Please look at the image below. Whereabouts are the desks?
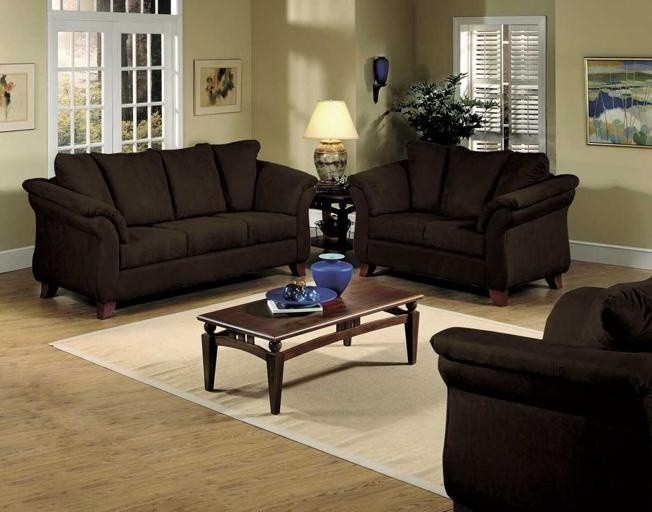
[310,195,356,260]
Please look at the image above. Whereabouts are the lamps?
[371,57,390,103]
[302,100,361,186]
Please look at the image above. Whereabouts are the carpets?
[49,280,543,502]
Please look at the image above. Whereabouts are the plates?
[266,286,338,304]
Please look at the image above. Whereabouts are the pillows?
[54,139,260,227]
[405,140,550,219]
[582,277,651,353]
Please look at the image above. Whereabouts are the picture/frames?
[0,62,38,132]
[582,55,652,150]
[191,59,243,116]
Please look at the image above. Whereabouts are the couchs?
[347,145,578,305]
[22,139,318,319]
[429,277,651,512]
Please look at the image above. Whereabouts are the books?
[267,300,324,315]
